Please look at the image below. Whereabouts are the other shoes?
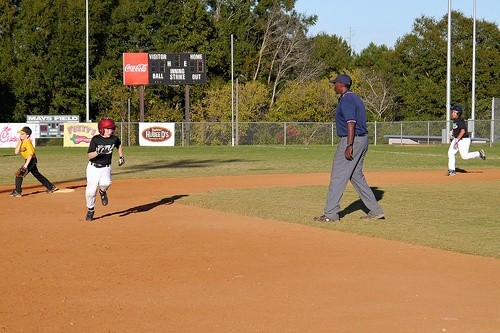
[479,149,486,160]
[313,215,335,222]
[445,169,456,176]
[9,190,22,197]
[47,186,58,194]
[359,211,385,221]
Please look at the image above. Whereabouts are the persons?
[446,106,486,176]
[85,117,125,221]
[313,75,385,222]
[8,127,58,197]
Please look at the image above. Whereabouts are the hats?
[329,74,353,85]
[450,106,462,111]
[17,126,32,135]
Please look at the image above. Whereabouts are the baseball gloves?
[96,146,110,155]
[16,168,28,178]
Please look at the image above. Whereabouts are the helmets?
[98,118,118,136]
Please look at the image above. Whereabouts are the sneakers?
[86,207,95,222]
[99,189,108,206]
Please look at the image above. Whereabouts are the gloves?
[95,147,110,154]
[118,156,125,167]
[452,138,459,149]
[449,130,454,139]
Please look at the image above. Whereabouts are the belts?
[338,133,367,138]
[91,162,109,168]
